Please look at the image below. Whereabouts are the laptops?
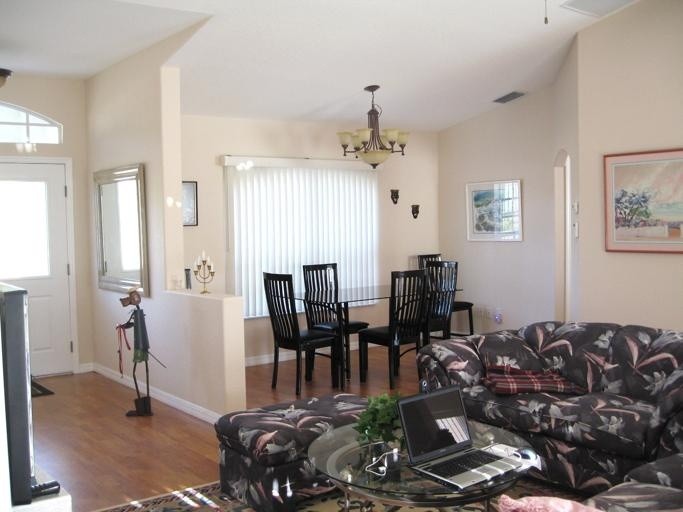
[395,383,522,492]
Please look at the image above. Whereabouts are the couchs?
[414,319,682,510]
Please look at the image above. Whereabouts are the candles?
[192,251,216,275]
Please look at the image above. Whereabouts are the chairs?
[260,251,473,397]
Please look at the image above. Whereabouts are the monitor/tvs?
[0,281,37,505]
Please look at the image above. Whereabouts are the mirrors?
[94,164,148,297]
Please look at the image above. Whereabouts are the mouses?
[512,447,538,462]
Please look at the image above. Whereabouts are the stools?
[214,391,400,512]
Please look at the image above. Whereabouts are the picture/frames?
[180,180,199,227]
[466,179,523,243]
[603,151,682,256]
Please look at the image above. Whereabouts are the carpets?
[31,381,54,399]
[99,450,594,512]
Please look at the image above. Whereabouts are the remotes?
[32,481,62,498]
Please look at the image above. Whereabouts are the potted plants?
[356,393,401,444]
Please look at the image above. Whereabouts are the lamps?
[338,85,411,168]
